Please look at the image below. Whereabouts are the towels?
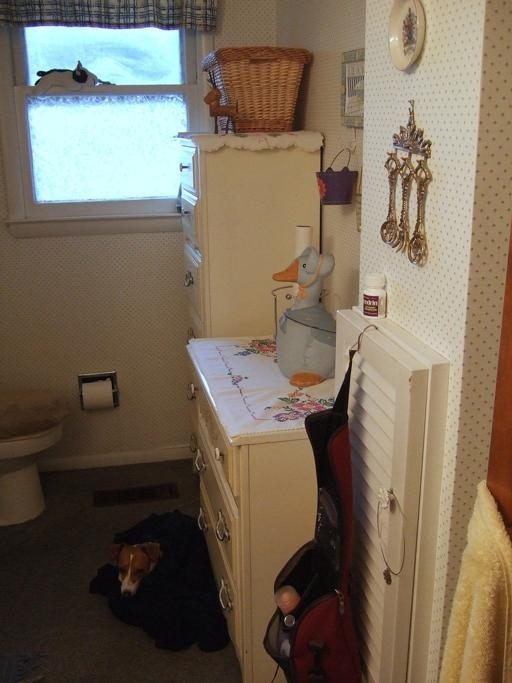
[438,479,511,682]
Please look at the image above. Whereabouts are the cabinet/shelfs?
[185,338,334,682]
[175,132,325,346]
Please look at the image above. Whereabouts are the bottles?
[276,587,300,615]
[362,273,387,317]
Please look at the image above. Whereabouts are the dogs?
[110,541,162,597]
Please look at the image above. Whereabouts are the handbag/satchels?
[263,410,363,681]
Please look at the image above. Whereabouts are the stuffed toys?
[270,245,335,389]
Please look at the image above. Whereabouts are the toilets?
[0,392,62,528]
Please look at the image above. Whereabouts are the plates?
[387,0,425,71]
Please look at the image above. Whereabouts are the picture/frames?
[341,48,364,128]
